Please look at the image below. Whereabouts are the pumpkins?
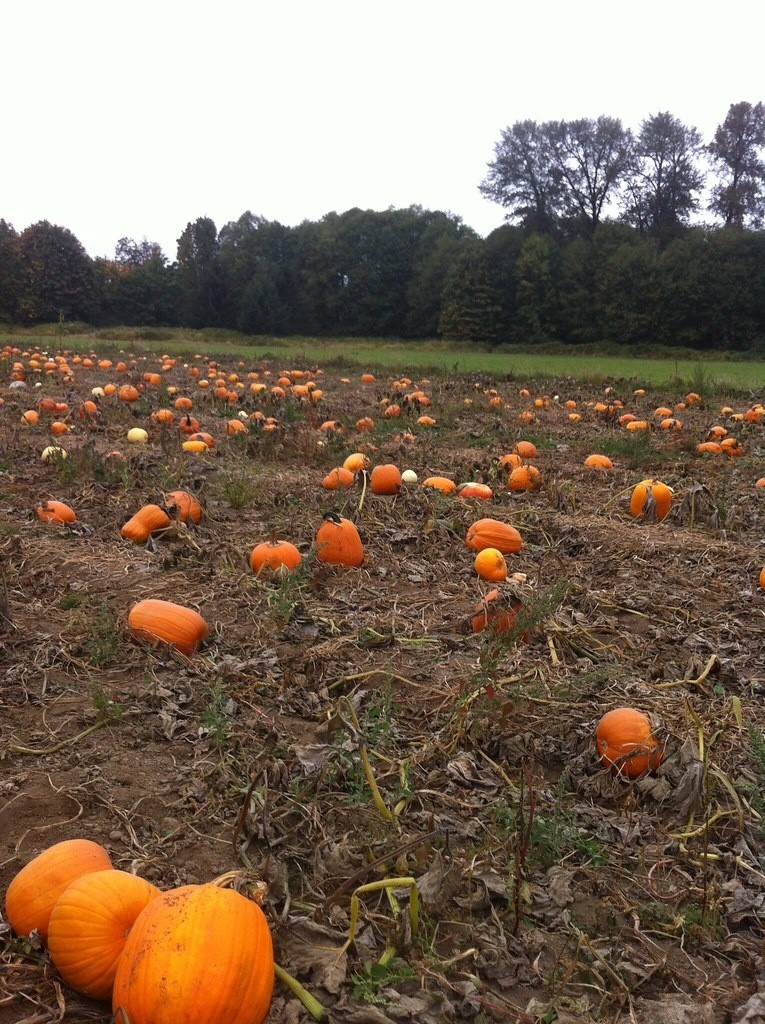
[597,708,662,779]
[472,589,533,644]
[5,838,115,946]
[129,598,208,655]
[0,346,765,589]
[48,870,162,998]
[112,870,274,1023]
